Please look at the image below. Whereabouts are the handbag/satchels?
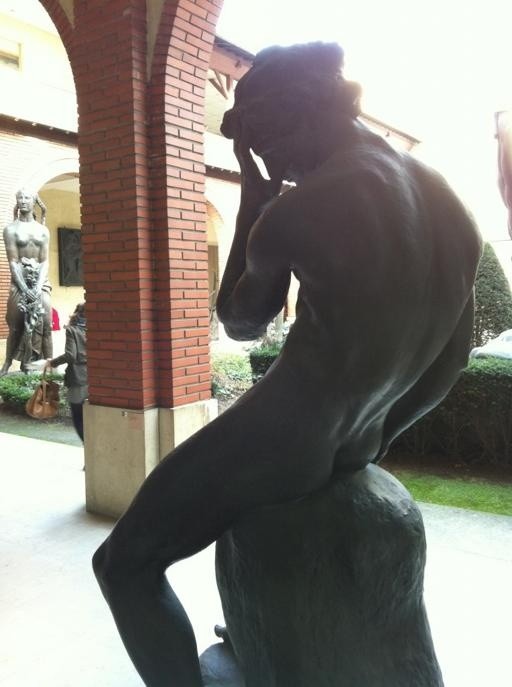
[26,381,61,419]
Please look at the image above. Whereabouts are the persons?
[47,304,87,442]
[50,307,60,331]
[1,186,54,377]
[89,39,487,686]
